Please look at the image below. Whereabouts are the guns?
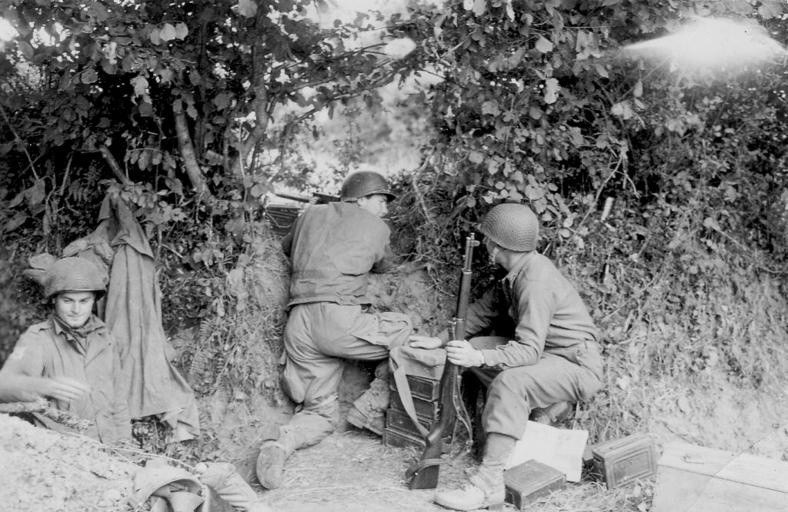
[276,192,340,204]
[409,232,481,489]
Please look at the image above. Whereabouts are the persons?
[254,170,413,490]
[0,257,132,451]
[406,202,603,512]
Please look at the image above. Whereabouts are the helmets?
[476,203,539,253]
[43,257,107,302]
[341,172,399,202]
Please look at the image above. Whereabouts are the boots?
[534,402,572,427]
[346,378,392,437]
[256,433,303,492]
[434,433,516,512]
